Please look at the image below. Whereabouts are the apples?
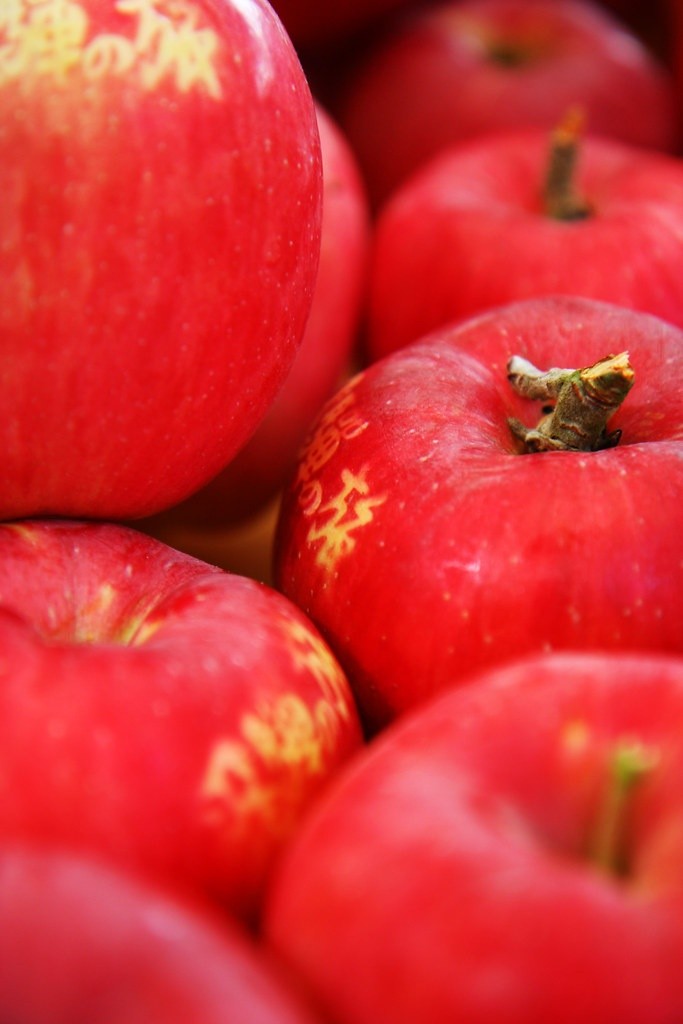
[0,0,683,1024]
